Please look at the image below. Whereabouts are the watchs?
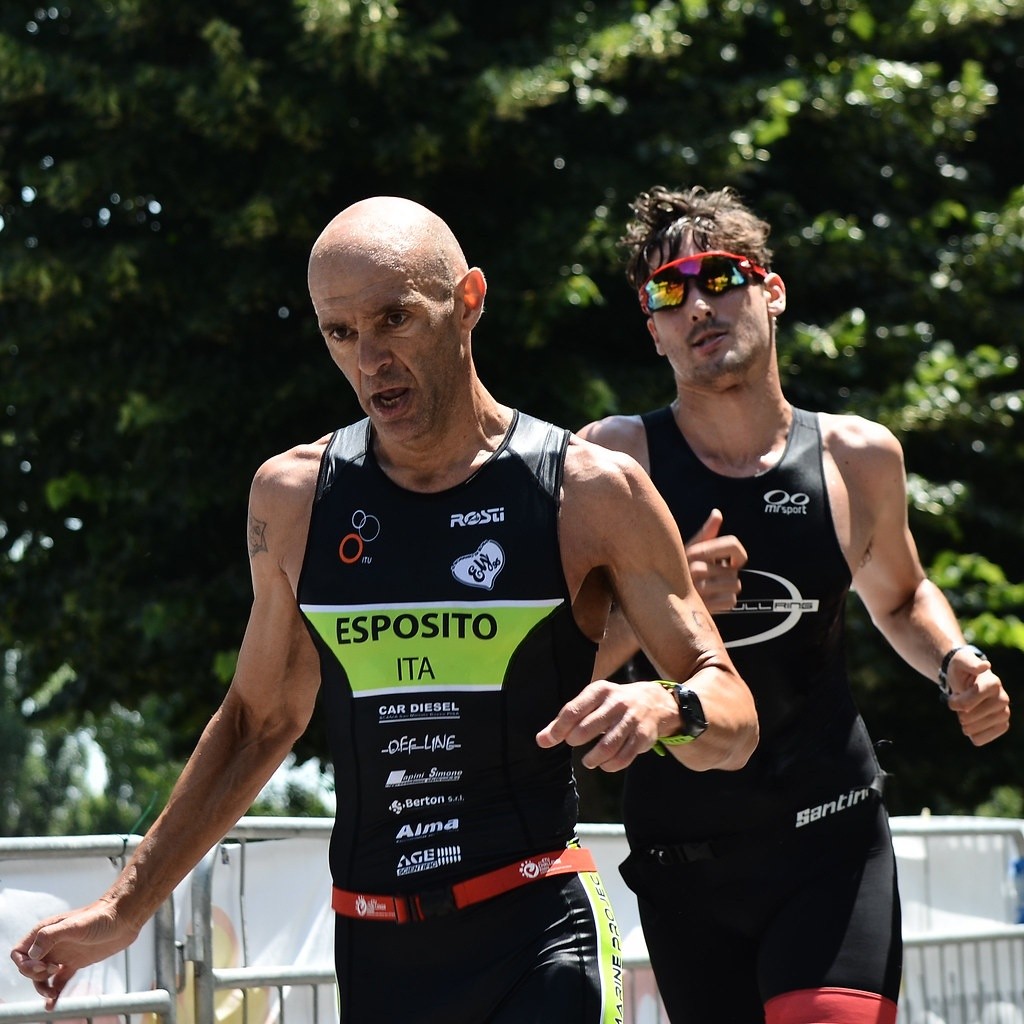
[652,680,708,756]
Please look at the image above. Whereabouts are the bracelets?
[938,646,987,697]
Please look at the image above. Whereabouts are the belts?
[333,849,598,925]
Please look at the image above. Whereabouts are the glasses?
[638,251,767,314]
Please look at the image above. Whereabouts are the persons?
[10,197,758,1024]
[568,189,1011,1024]
